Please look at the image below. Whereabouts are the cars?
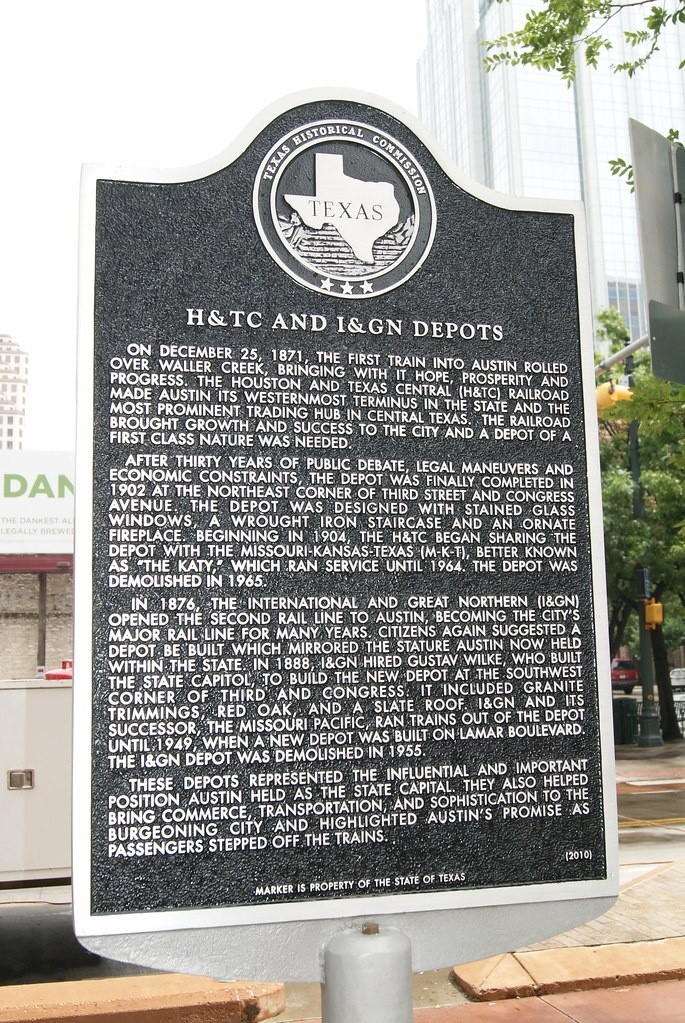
[669,667,685,692]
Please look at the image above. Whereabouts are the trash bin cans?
[613,696,638,743]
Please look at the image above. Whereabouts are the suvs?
[610,658,639,695]
